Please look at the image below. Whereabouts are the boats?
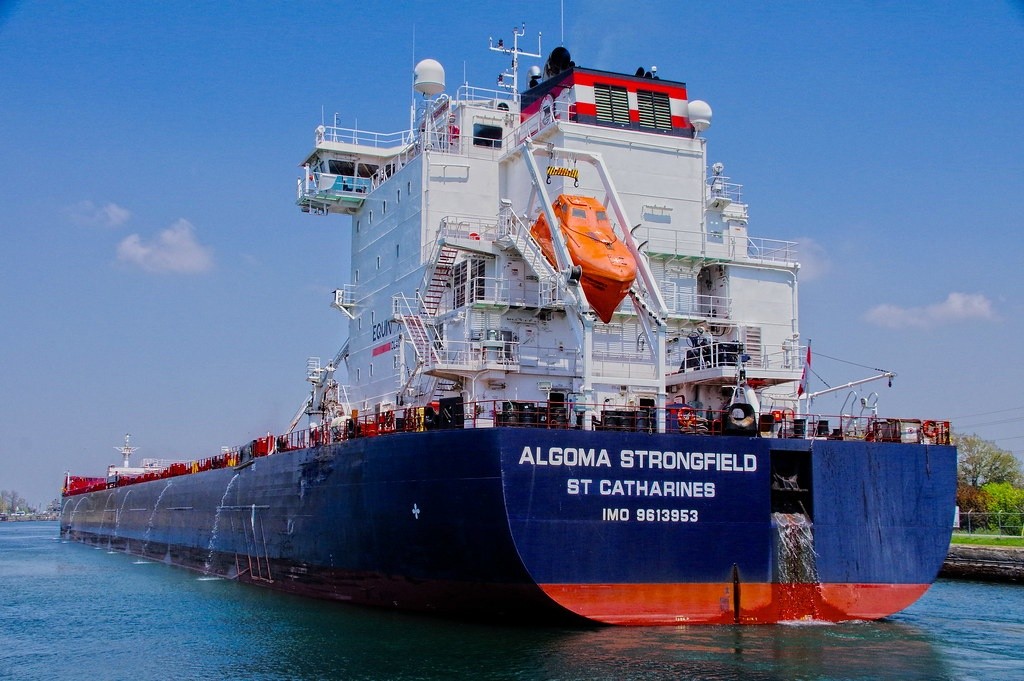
[59,0,960,628]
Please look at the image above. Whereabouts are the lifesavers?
[923,420,939,437]
[677,407,695,426]
[386,410,394,427]
